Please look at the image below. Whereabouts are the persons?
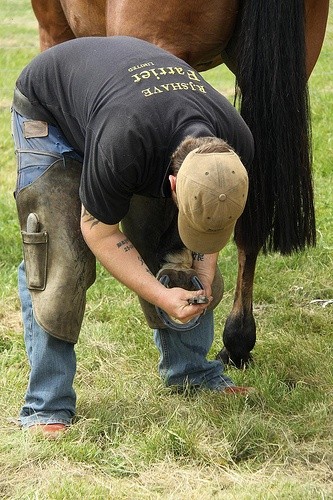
[7,34,263,445]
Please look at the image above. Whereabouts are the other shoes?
[184,383,258,408]
[21,418,74,442]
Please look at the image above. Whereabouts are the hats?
[177,145,250,254]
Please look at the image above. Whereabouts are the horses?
[30,0,328,370]
